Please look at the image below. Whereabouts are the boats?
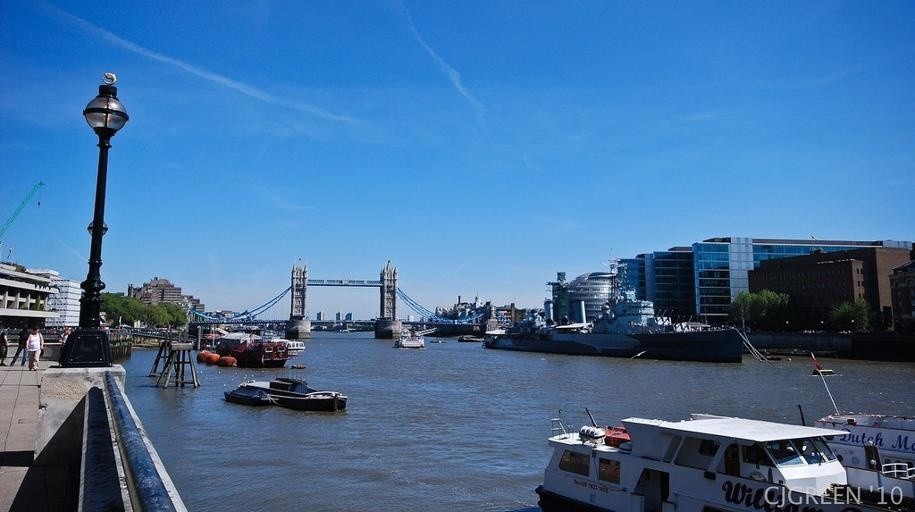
[224,377,346,412]
[392,330,424,348]
[187,319,306,368]
[813,368,834,375]
[744,348,838,361]
[458,336,485,341]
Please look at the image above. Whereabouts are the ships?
[481,248,743,362]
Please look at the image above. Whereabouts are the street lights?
[57,71,130,331]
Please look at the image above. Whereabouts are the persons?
[10,325,30,366]
[26,327,44,370]
[0,325,11,366]
[5,327,76,343]
[99,323,123,342]
